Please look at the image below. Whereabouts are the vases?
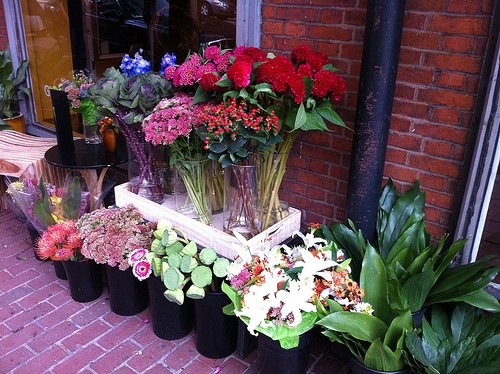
[27,228,51,261]
[257,332,312,374]
[62,260,104,303]
[151,274,195,341]
[175,158,213,227]
[256,151,289,229]
[128,141,165,204]
[196,292,238,358]
[349,355,411,374]
[106,265,151,317]
[55,262,68,280]
[83,116,103,144]
[223,163,260,239]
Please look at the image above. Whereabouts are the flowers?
[6,40,500,374]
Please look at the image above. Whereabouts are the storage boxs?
[113,183,302,261]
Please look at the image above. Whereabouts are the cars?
[90,0,236,60]
[199,0,237,20]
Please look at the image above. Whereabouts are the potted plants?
[0,50,34,136]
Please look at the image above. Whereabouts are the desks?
[45,139,135,209]
